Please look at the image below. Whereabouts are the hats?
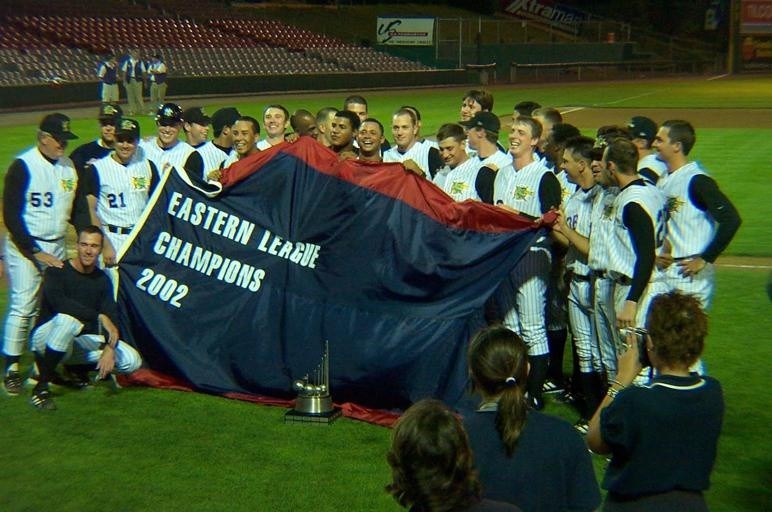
[97,101,123,120]
[105,53,115,59]
[212,107,240,125]
[624,116,658,141]
[180,106,212,124]
[458,111,500,132]
[152,54,160,59]
[113,119,139,138]
[40,114,78,140]
[154,102,183,126]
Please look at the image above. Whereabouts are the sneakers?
[149,110,156,116]
[542,380,566,394]
[31,389,51,408]
[1,371,22,396]
[128,109,135,117]
[66,371,95,391]
[552,391,579,403]
[137,109,146,115]
[28,362,66,386]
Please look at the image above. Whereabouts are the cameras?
[618,327,648,355]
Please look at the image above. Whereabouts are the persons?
[121,49,147,116]
[453,326,603,512]
[98,53,121,106]
[652,119,742,376]
[31,225,143,410]
[587,288,724,511]
[2,112,90,397]
[68,89,670,434]
[147,55,167,115]
[385,399,520,510]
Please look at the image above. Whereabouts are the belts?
[573,273,589,282]
[104,82,116,85]
[616,278,633,286]
[673,258,686,262]
[107,225,132,234]
[150,80,157,83]
[129,77,135,79]
[597,272,606,278]
[29,235,66,244]
